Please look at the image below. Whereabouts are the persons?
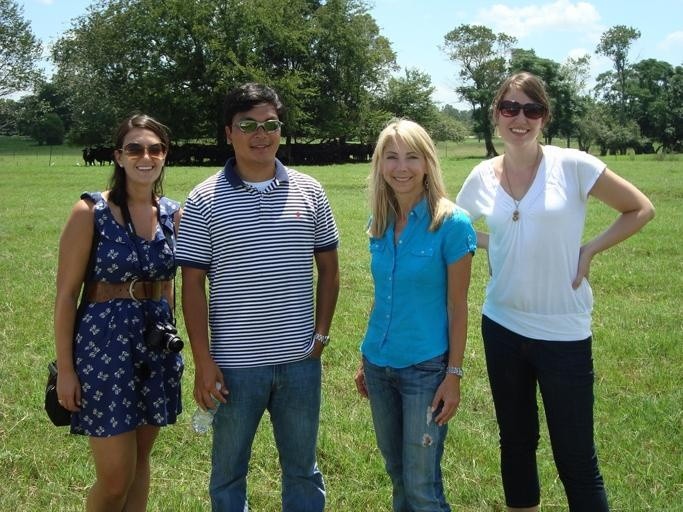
[454,73,654,511]
[175,85,338,512]
[54,112,182,512]
[351,119,478,511]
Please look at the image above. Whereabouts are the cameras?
[145,320,184,353]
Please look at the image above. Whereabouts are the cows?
[82,142,376,166]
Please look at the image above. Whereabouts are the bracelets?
[444,366,464,377]
[314,332,332,346]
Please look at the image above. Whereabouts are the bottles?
[191,381,220,434]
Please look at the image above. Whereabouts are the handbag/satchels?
[44,360,72,426]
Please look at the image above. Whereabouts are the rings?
[58,398,65,404]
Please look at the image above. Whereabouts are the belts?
[84,279,172,304]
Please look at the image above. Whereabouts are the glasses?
[234,119,284,133]
[498,99,548,119]
[119,142,168,160]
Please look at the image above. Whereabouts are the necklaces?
[502,146,541,221]
[237,166,276,180]
